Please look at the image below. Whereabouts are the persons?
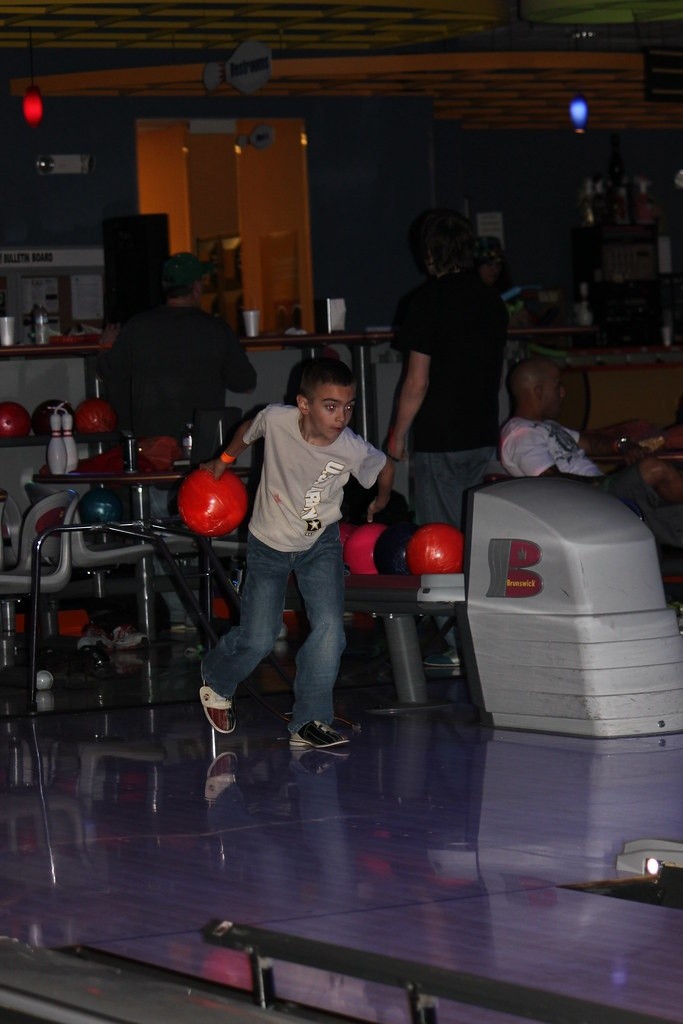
[94,252,257,634]
[498,356,683,552]
[472,237,523,307]
[386,209,510,669]
[203,747,352,918]
[197,354,393,748]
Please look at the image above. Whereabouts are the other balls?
[0,402,32,439]
[80,488,125,526]
[32,399,75,435]
[74,398,117,432]
[405,523,465,577]
[178,464,250,538]
[374,521,418,576]
[343,524,386,575]
[337,523,355,546]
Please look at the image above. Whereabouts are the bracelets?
[219,451,236,465]
[622,441,635,453]
[617,437,629,449]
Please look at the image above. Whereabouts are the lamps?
[567,88,590,135]
[22,28,45,130]
[35,152,95,177]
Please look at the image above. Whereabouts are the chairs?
[25,480,158,602]
[0,488,22,633]
[0,488,79,640]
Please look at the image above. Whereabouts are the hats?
[469,237,504,261]
[161,252,217,286]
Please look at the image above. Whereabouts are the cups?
[244,311,260,337]
[0,316,15,346]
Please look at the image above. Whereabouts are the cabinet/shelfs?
[572,223,664,346]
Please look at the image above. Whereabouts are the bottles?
[36,303,49,345]
[183,423,195,459]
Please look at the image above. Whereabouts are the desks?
[0,324,593,445]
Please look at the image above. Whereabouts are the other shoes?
[424,648,461,667]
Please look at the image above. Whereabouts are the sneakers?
[112,624,149,648]
[200,661,236,734]
[76,624,113,651]
[289,722,350,749]
